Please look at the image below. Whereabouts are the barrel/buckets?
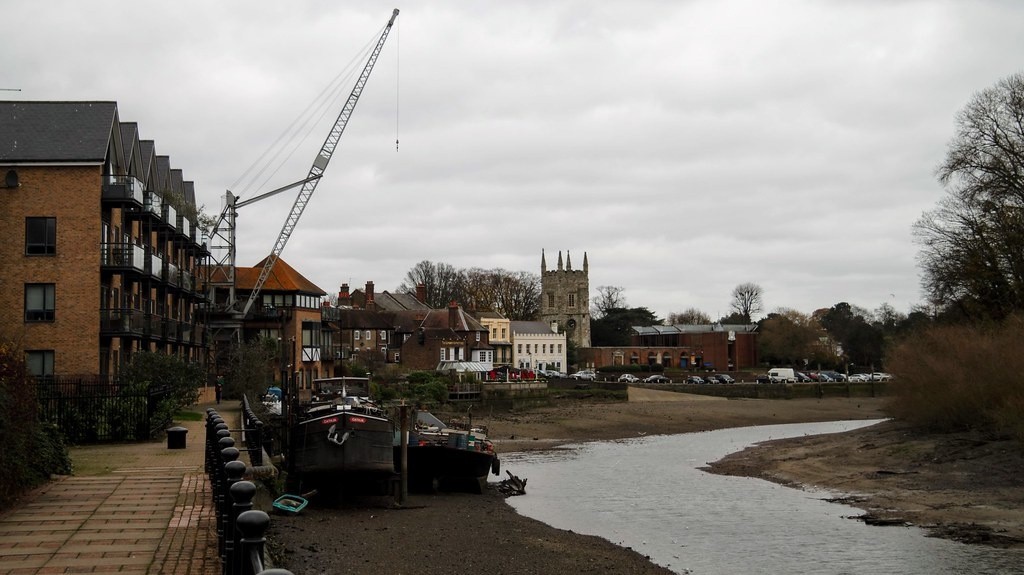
[408,433,418,447]
[447,432,475,450]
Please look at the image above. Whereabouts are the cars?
[533,370,569,379]
[755,375,778,384]
[685,376,704,384]
[820,374,833,382]
[795,372,811,382]
[842,373,877,383]
[619,375,640,383]
[643,375,670,384]
[703,376,720,384]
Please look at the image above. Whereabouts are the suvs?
[571,371,597,381]
[715,374,735,384]
[870,373,889,381]
[801,371,819,382]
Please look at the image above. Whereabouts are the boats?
[393,404,495,490]
[263,388,282,417]
[282,301,394,499]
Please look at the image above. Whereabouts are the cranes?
[205,7,398,381]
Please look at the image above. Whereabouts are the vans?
[820,371,846,382]
[768,368,795,383]
